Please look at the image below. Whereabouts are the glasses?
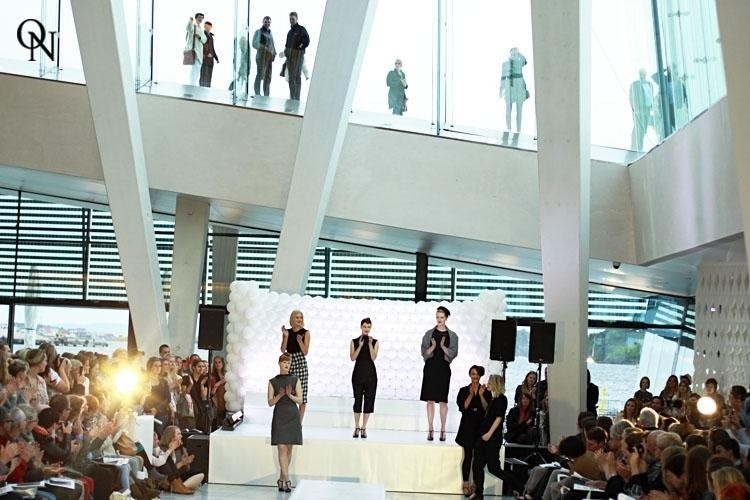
[7,419,15,423]
[395,62,401,64]
[67,408,73,412]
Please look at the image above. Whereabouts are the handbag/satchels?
[183,24,195,65]
[525,84,529,99]
[129,471,160,500]
[115,432,137,456]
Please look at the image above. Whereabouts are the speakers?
[529,322,556,364]
[490,319,517,362]
[198,307,225,351]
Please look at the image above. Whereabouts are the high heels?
[353,427,360,437]
[467,491,484,500]
[285,480,292,493]
[439,431,446,441]
[427,430,435,441]
[277,479,284,492]
[361,429,367,438]
[463,486,472,498]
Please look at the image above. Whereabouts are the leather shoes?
[178,478,195,491]
[158,480,171,491]
[171,479,195,495]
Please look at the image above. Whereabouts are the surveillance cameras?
[613,262,622,269]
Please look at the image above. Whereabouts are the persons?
[613,373,750,421]
[464,374,524,500]
[127,343,227,432]
[629,69,653,152]
[0,340,127,410]
[350,318,378,437]
[499,48,530,135]
[652,63,689,140]
[536,367,548,400]
[455,366,490,496]
[252,16,277,95]
[0,407,162,499]
[586,370,599,414]
[507,393,536,443]
[200,22,219,87]
[183,13,207,88]
[268,353,303,492]
[279,12,309,98]
[514,372,537,405]
[281,311,311,422]
[386,60,409,116]
[153,434,195,495]
[159,425,205,489]
[419,306,458,441]
[512,412,750,500]
